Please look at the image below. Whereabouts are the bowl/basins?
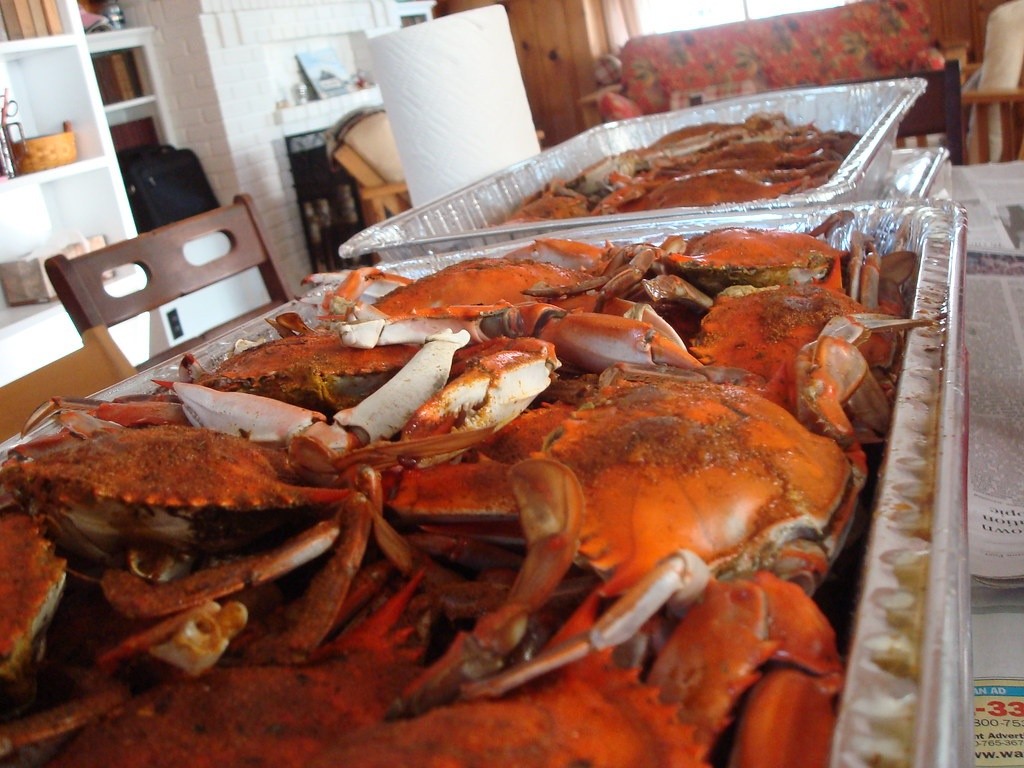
[13,120,76,173]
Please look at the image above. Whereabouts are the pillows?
[601,91,641,119]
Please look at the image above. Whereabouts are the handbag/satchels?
[125,144,222,236]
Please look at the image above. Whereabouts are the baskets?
[5,120,78,174]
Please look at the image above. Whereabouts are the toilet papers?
[359,4,543,211]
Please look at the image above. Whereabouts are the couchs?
[597,0,972,123]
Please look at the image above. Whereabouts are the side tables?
[579,84,622,128]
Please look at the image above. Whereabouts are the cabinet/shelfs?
[0,0,150,386]
[284,126,374,273]
[85,26,180,150]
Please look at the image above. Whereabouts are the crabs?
[0,209,943,768]
[486,110,865,227]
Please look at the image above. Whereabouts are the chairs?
[824,58,964,165]
[0,325,137,444]
[44,193,291,372]
[325,105,546,263]
